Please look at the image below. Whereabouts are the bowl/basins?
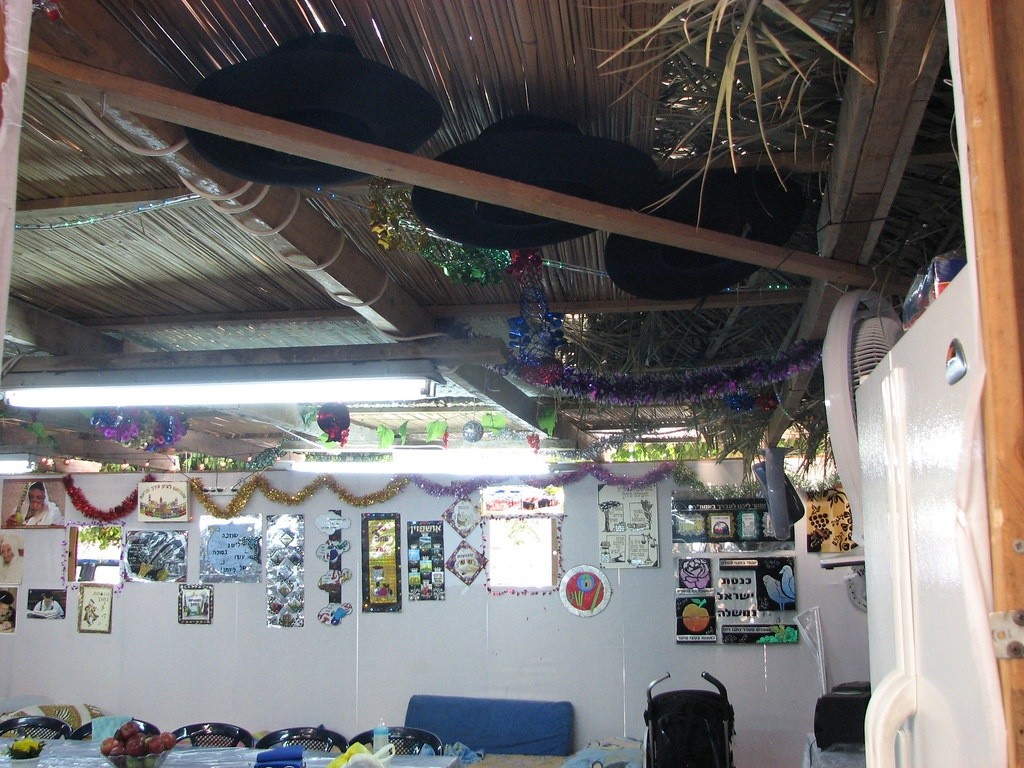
[98,749,173,768]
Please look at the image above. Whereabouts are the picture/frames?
[361,512,402,613]
[178,583,214,624]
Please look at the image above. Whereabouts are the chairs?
[404,694,573,754]
[0,714,445,754]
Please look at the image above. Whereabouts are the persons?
[0,590,16,633]
[6,481,65,526]
[0,534,22,580]
[34,591,64,615]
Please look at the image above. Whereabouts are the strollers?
[642,673,736,768]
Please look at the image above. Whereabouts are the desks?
[0,738,459,768]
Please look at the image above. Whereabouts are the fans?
[823,289,905,564]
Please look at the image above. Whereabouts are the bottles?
[373,718,389,768]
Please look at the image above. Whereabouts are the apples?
[101,722,176,768]
